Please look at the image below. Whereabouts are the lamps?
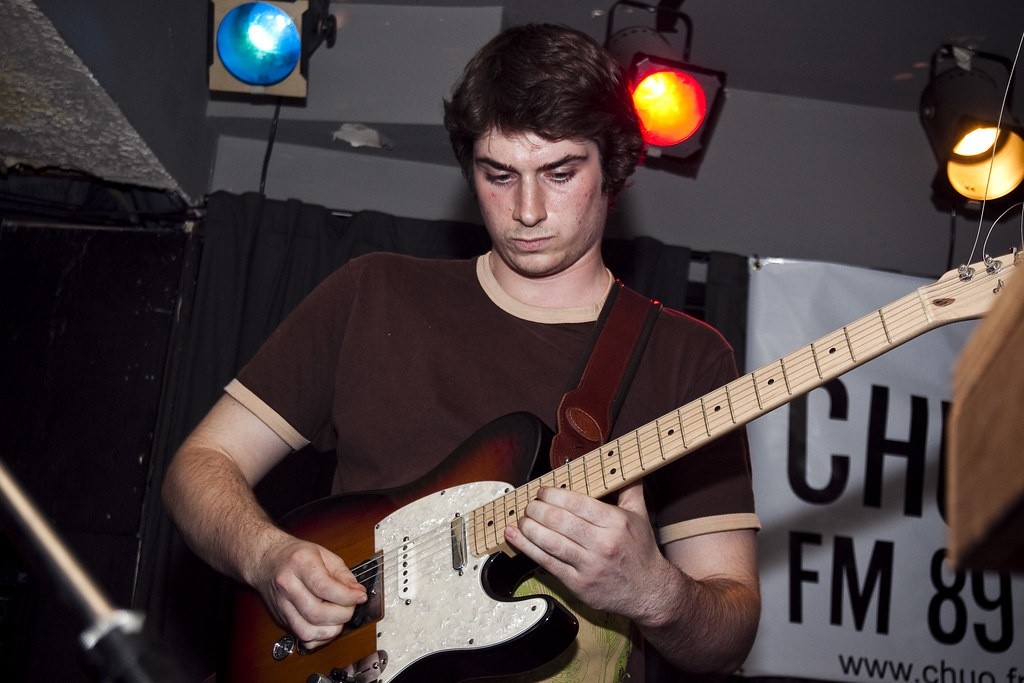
[918,43,1024,221]
[603,0,728,180]
[208,0,336,98]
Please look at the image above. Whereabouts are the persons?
[164,24,763,683]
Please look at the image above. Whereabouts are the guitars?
[235,245,1018,683]
